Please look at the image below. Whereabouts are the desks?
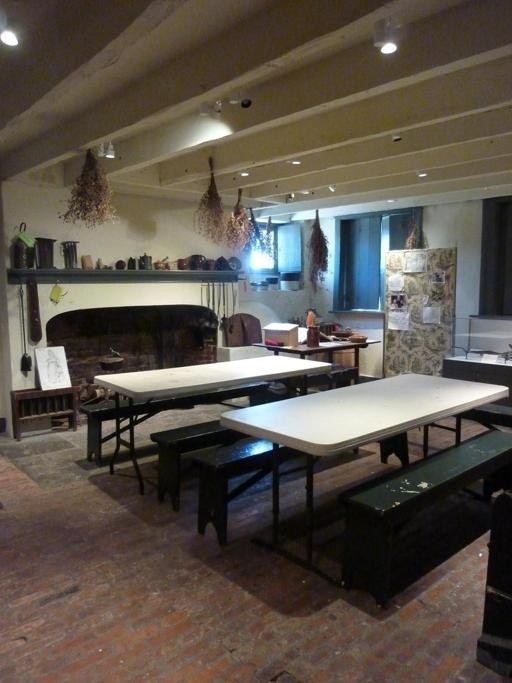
[251,337,380,395]
[218,371,511,583]
[92,354,334,500]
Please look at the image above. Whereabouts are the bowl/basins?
[154,261,168,270]
[348,335,368,343]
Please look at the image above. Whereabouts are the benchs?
[76,384,512,595]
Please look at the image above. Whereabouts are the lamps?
[372,15,399,57]
[96,141,116,158]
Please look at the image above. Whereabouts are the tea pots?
[137,252,153,270]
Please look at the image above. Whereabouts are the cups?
[190,254,207,270]
[207,259,216,271]
[176,258,189,269]
